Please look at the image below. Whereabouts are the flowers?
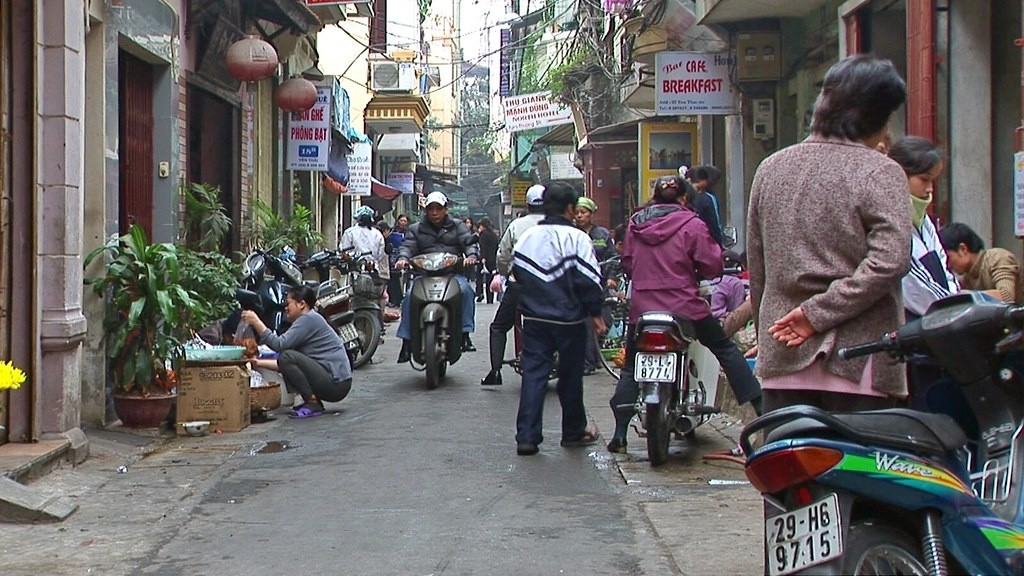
[0,361,27,430]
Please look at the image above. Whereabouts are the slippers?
[561,425,598,448]
[516,442,539,456]
[287,408,322,418]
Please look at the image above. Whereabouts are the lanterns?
[225,35,278,84]
[273,75,318,115]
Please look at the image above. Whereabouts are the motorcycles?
[223,239,386,372]
[498,264,563,381]
[737,289,1023,575]
[384,234,480,389]
[603,226,743,466]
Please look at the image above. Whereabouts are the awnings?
[427,170,458,181]
[427,177,463,195]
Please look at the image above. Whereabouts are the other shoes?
[397,348,410,363]
[607,435,628,453]
[480,370,503,385]
[463,339,476,351]
[477,294,484,302]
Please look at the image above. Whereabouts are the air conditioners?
[370,62,418,90]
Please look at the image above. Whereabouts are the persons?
[198,308,242,345]
[338,54,1019,457]
[241,285,352,418]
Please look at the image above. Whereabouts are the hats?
[425,191,448,206]
[525,184,546,205]
[377,220,388,227]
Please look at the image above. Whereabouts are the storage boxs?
[175,364,252,435]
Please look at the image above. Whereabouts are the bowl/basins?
[181,421,211,437]
[184,345,246,360]
[602,348,620,361]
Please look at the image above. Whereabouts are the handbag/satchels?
[490,274,503,293]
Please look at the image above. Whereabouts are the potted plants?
[83,223,220,428]
